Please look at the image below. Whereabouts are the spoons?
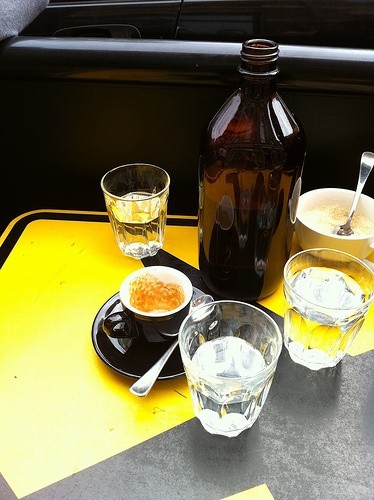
[129,294,215,397]
[334,152,374,236]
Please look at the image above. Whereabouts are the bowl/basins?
[103,265,194,353]
[295,188,374,262]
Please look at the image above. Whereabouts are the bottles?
[197,39,305,302]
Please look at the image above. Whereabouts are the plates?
[91,286,222,382]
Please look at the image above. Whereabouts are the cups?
[178,300,282,438]
[283,248,374,372]
[100,163,170,260]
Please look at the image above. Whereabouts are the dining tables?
[0,209,374,500]
